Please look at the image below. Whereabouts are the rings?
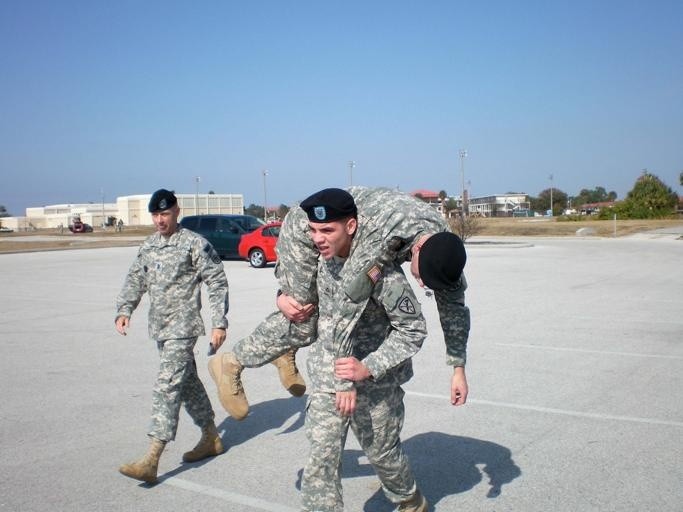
[345,399,350,402]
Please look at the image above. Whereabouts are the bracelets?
[453,365,466,369]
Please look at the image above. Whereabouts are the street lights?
[262,169,268,223]
[194,176,199,215]
[347,160,355,186]
[457,149,467,219]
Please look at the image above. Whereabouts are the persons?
[206,185,469,421]
[276,188,431,510]
[117,218,123,232]
[112,187,229,482]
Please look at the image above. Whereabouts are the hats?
[149,189,177,213]
[300,189,358,223]
[417,232,467,290]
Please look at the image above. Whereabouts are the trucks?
[101,216,116,226]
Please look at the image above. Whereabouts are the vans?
[179,214,267,262]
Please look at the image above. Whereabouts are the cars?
[238,222,282,268]
[0,227,14,233]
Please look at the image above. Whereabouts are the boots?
[118,439,167,484]
[183,424,224,461]
[273,349,307,398]
[208,349,251,420]
